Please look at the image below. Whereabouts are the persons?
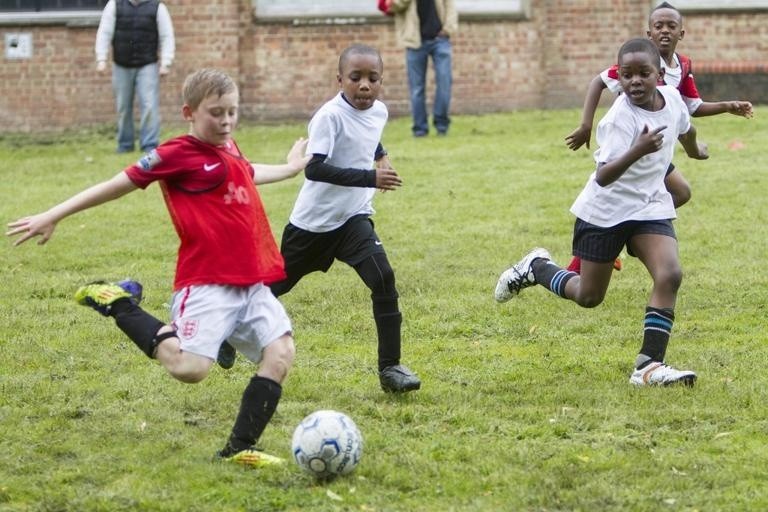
[5,69,314,468]
[564,2,754,273]
[218,45,421,394]
[493,37,710,389]
[95,1,176,152]
[378,1,459,135]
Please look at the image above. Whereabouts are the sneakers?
[567,255,582,272]
[629,361,698,387]
[216,341,236,369]
[212,445,289,469]
[494,247,551,302]
[379,364,421,395]
[73,277,143,317]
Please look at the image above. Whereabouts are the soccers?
[291,410,363,479]
